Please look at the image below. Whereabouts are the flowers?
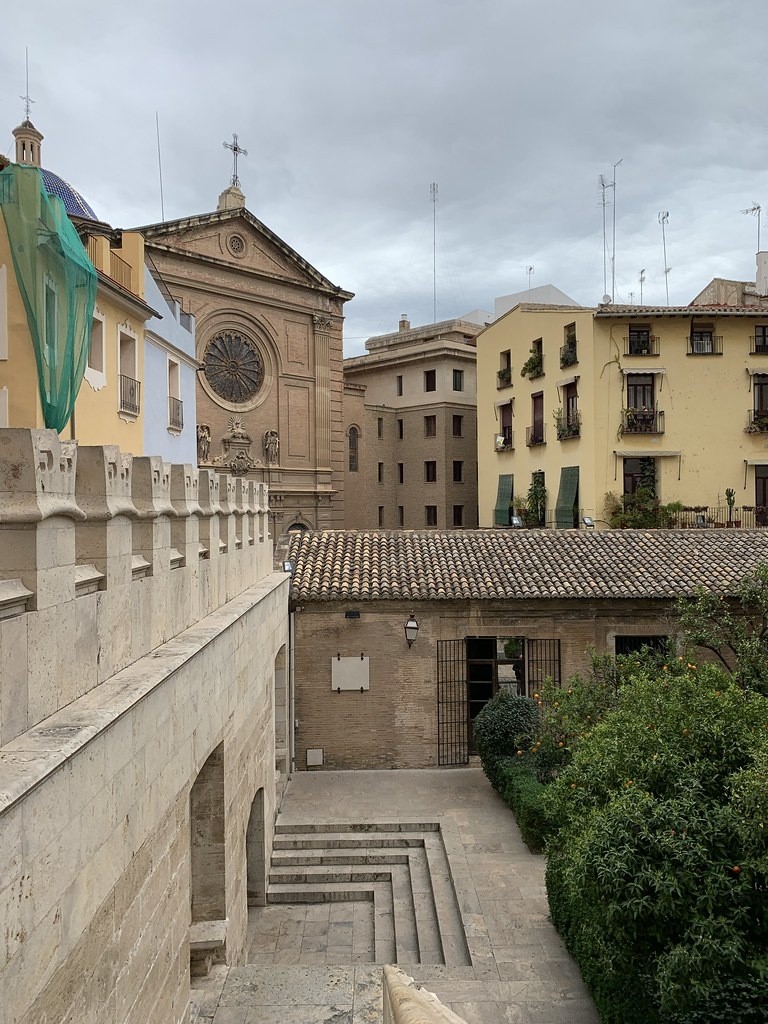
[618,406,654,427]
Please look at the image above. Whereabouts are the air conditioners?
[694,341,712,353]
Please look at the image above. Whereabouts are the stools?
[188,919,227,976]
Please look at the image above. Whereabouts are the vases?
[641,427,646,432]
[651,425,657,432]
[627,428,630,431]
[637,429,640,431]
[632,429,635,432]
[650,335,655,341]
[646,428,650,432]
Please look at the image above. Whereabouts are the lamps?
[282,559,295,573]
[511,516,524,530]
[583,517,595,529]
[344,611,362,620]
[403,610,419,649]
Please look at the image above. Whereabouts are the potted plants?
[751,416,768,431]
[552,407,582,439]
[733,508,742,528]
[724,487,736,528]
[714,491,726,528]
[642,345,648,354]
[497,369,511,386]
[507,495,528,516]
